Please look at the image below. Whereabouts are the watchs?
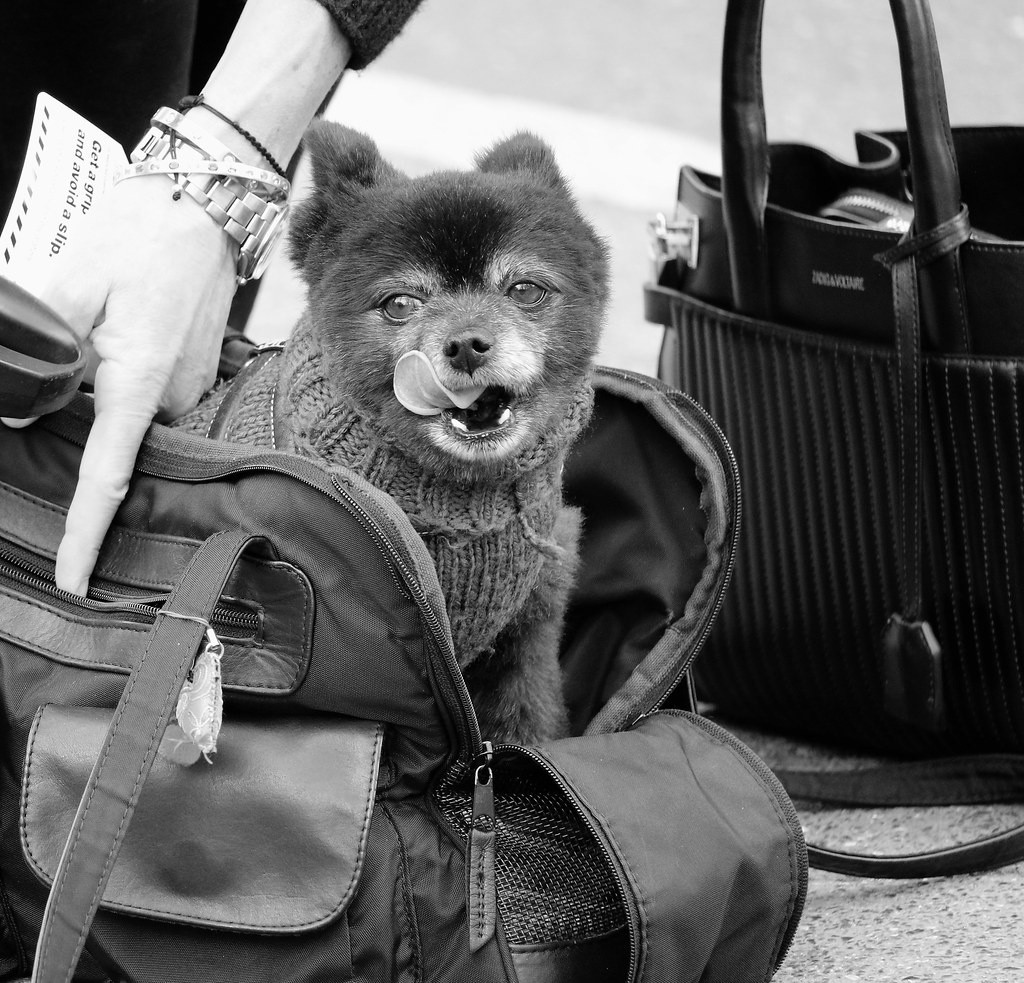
[130,106,289,287]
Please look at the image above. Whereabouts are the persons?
[0,0,426,600]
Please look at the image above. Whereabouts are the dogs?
[153,113,614,756]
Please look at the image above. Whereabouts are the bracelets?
[111,96,291,198]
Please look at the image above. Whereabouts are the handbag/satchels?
[0,329,808,983]
[645,1,1023,763]
